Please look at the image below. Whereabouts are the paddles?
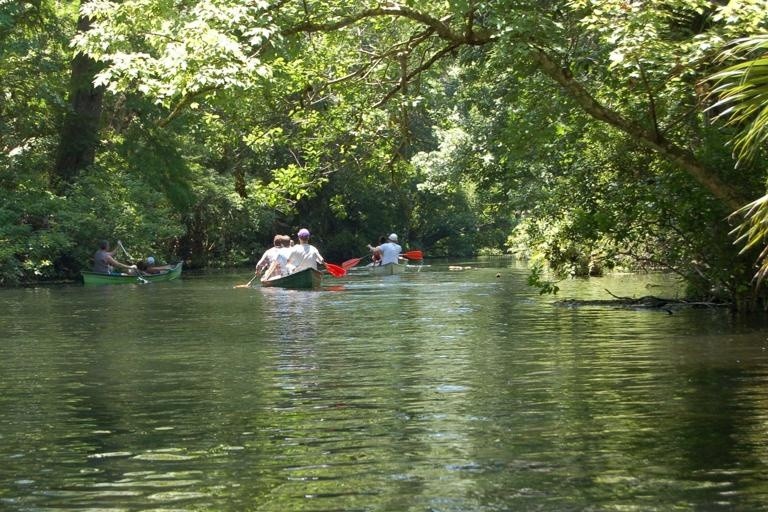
[342,252,373,269]
[324,262,346,278]
[121,243,151,284]
[398,250,423,260]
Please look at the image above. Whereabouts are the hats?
[297,228,311,238]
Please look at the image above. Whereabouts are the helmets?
[146,257,155,265]
[99,239,110,249]
[388,233,398,242]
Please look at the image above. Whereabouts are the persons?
[129,256,172,273]
[371,236,387,262]
[93,239,136,275]
[365,231,403,265]
[126,260,149,276]
[254,227,328,284]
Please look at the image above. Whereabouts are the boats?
[367,260,407,275]
[80,260,184,286]
[260,267,324,292]
